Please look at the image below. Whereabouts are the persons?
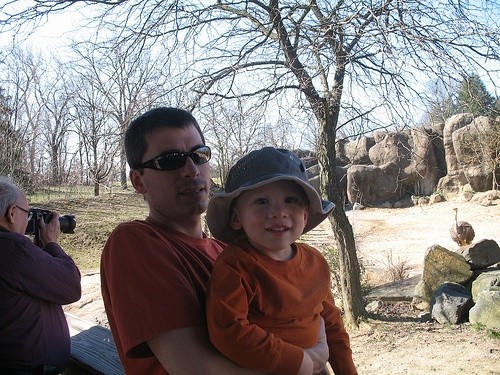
[0,178,82,375]
[100,107,328,375]
[206,147,357,375]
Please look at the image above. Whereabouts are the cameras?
[25,206,76,235]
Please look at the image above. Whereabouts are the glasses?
[136,145,212,170]
[10,204,33,216]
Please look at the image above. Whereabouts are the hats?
[206,146,336,244]
